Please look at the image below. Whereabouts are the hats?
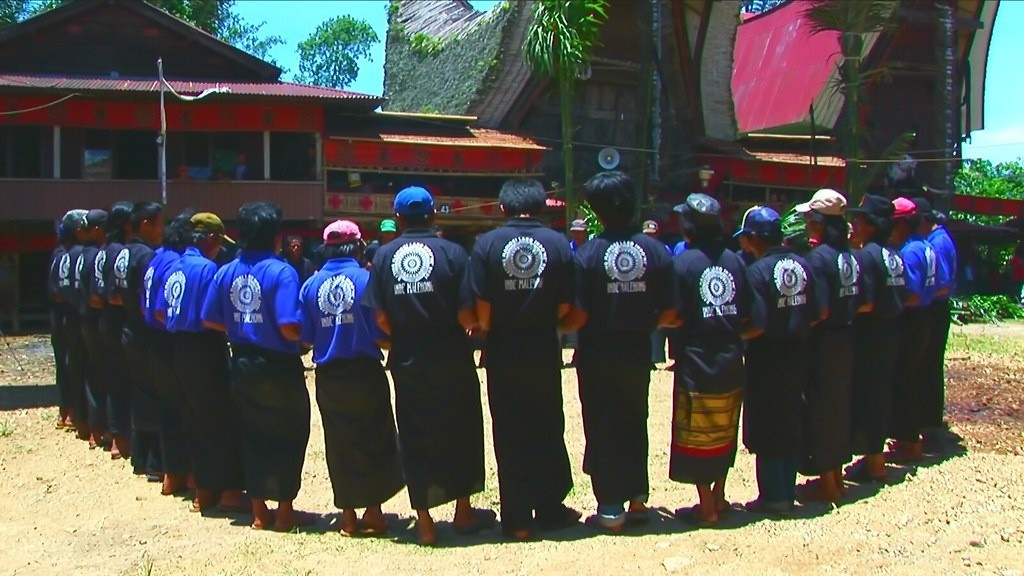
[846,192,895,219]
[795,188,849,215]
[379,218,397,232]
[733,204,781,239]
[570,219,587,231]
[323,219,367,247]
[642,220,659,234]
[189,212,236,244]
[892,197,933,218]
[81,207,108,230]
[393,186,435,217]
[674,192,721,216]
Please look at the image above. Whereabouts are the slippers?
[674,438,923,528]
[190,493,252,514]
[416,520,437,546]
[252,511,273,530]
[541,503,583,532]
[56,422,195,495]
[340,514,389,537]
[583,509,650,536]
[271,510,308,532]
[454,508,497,536]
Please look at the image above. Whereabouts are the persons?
[369,186,498,545]
[175,166,194,180]
[277,233,328,291]
[779,186,958,503]
[209,149,249,180]
[555,173,678,533]
[361,218,400,269]
[468,177,583,538]
[661,192,751,525]
[566,219,589,367]
[48,205,254,512]
[200,201,323,531]
[1009,246,1024,302]
[741,204,818,515]
[642,218,687,372]
[298,219,392,534]
[780,235,796,251]
